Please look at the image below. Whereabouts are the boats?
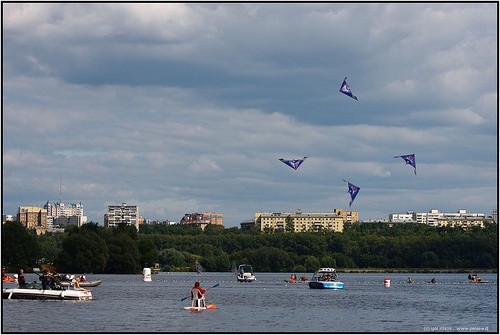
[32,281,103,288]
[307,267,344,289]
[235,264,256,282]
[3,287,93,301]
[284,275,307,283]
[183,304,217,312]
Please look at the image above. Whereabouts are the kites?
[392,153,417,177]
[341,178,361,208]
[277,155,308,171]
[337,76,360,102]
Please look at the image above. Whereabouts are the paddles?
[181,283,219,302]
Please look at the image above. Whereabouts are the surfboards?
[181,305,216,310]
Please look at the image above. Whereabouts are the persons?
[195,267,202,277]
[240,267,245,278]
[289,273,313,281]
[467,273,472,280]
[431,277,436,284]
[190,281,208,308]
[407,276,412,283]
[1,264,87,291]
[314,272,338,282]
